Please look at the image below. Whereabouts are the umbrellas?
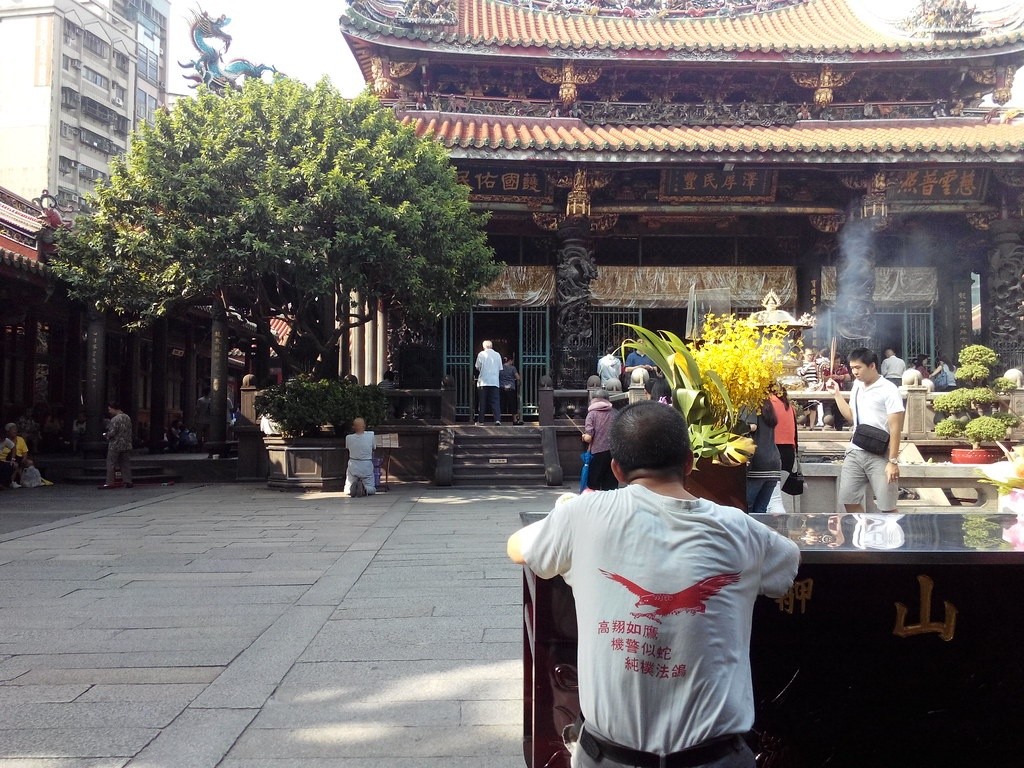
[580,434,591,494]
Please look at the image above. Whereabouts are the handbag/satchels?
[780,472,804,495]
[934,365,948,392]
[504,384,512,391]
[852,424,890,455]
[20,466,42,488]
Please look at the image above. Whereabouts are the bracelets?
[11,458,16,461]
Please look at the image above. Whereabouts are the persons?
[507,400,801,768]
[474,340,501,425]
[378,371,395,389]
[0,387,239,489]
[499,353,524,425]
[344,417,377,497]
[580,337,957,515]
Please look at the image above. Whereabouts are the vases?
[683,455,749,515]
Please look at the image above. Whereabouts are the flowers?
[610,301,808,471]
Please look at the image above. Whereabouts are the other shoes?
[495,421,502,425]
[350,480,363,498]
[10,482,21,489]
[513,420,524,425]
[479,423,484,426]
[97,484,115,489]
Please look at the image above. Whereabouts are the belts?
[580,728,740,768]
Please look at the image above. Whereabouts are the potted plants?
[932,344,1020,462]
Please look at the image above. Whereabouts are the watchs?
[889,458,898,464]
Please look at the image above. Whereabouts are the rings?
[895,476,898,479]
